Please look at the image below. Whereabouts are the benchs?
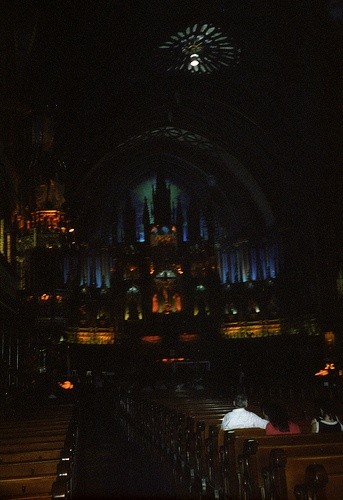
[117,375,343,500]
[0,395,80,499]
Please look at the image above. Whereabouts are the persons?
[261,399,302,436]
[310,400,343,433]
[220,392,270,430]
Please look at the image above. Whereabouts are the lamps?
[190,53,200,66]
